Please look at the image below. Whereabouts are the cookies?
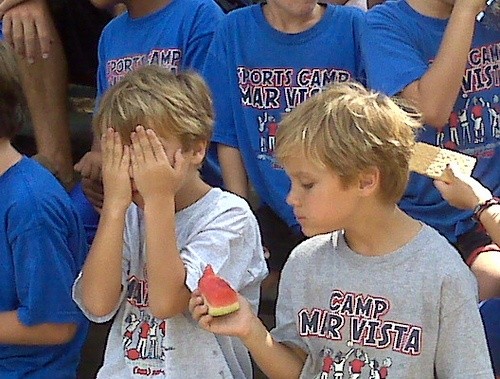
[408,142,477,183]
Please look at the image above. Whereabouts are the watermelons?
[197,264,240,316]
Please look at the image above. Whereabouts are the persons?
[1,0,127,190]
[187,82,495,379]
[0,72,90,379]
[72,65,270,379]
[76,1,500,379]
